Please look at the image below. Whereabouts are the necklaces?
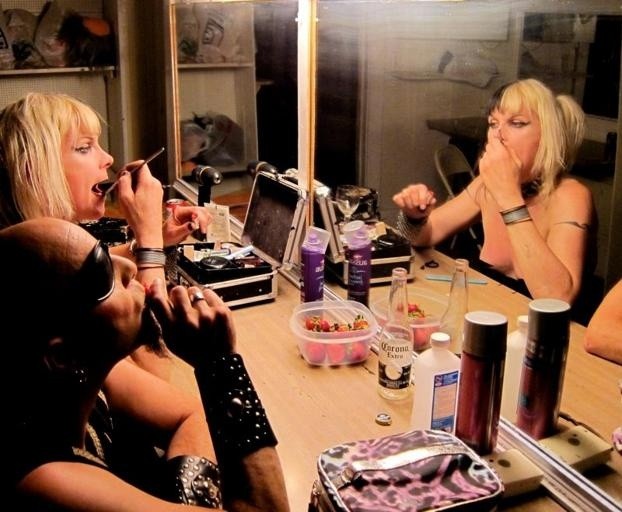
[512,177,544,201]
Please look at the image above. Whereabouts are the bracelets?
[497,204,534,226]
[157,454,223,510]
[191,351,280,463]
[134,244,168,270]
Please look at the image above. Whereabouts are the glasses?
[81,240,116,303]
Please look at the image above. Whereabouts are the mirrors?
[162,1,621,512]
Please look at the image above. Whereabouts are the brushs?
[100,146,169,197]
[497,129,504,145]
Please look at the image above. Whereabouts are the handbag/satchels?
[305,428,503,512]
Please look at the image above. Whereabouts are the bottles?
[500,314,528,425]
[514,298,571,441]
[377,266,416,401]
[439,258,472,355]
[413,332,462,439]
[343,220,371,310]
[454,310,508,457]
[299,225,331,320]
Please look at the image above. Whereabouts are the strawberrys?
[306,315,369,364]
[408,304,440,351]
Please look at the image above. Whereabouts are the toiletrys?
[204,202,233,244]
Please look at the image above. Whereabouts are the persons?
[392,74,596,314]
[0,214,294,512]
[583,275,622,368]
[0,88,219,311]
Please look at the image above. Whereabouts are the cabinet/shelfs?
[174,2,260,211]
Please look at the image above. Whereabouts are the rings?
[188,292,206,303]
